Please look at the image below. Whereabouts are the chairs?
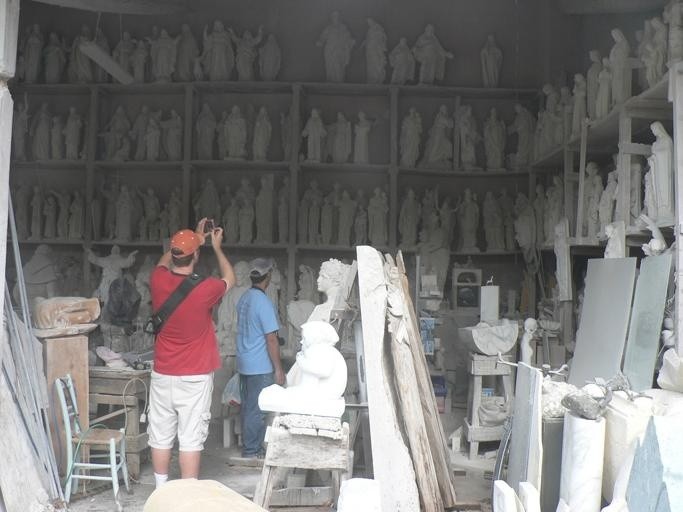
[54,373,133,511]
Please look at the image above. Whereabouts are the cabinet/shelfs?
[9,66,683,354]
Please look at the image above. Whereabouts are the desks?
[89,366,150,482]
[464,352,513,459]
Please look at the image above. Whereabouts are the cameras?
[134,361,151,370]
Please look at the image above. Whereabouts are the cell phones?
[205,219,214,233]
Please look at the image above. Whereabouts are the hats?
[249,257,273,278]
[170,228,206,258]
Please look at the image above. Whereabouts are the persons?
[103,105,183,160]
[389,37,415,85]
[194,176,289,243]
[24,24,46,82]
[400,103,536,171]
[480,35,503,88]
[298,182,388,247]
[315,11,352,83]
[15,186,85,240]
[362,18,387,84]
[399,188,528,250]
[94,183,182,240]
[230,258,285,459]
[256,320,348,418]
[15,92,83,161]
[519,317,538,366]
[308,258,351,319]
[63,26,177,84]
[176,20,281,80]
[534,122,675,243]
[197,102,272,161]
[412,23,454,87]
[145,216,237,488]
[302,108,371,164]
[82,245,139,302]
[43,32,66,83]
[534,3,682,161]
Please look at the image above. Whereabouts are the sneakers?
[241,447,266,459]
